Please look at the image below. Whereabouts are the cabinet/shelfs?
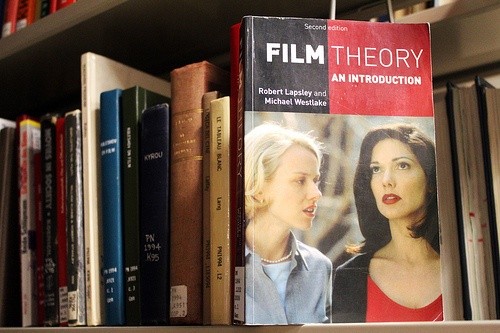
[0,0,500,333]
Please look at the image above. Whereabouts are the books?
[0,0,500,327]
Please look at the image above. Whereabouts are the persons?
[333,125,443,321]
[243,121,332,323]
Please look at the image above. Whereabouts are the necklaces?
[262,250,293,263]
